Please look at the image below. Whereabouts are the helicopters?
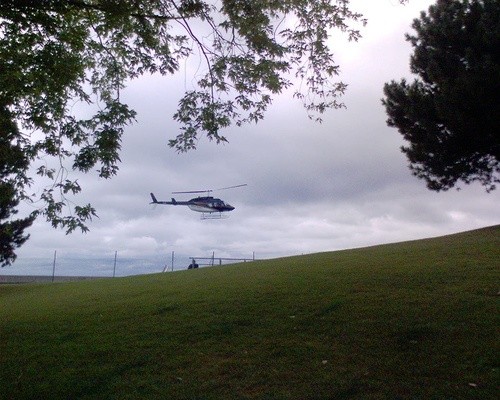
[148,184,248,219]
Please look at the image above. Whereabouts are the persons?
[187,260,198,270]
